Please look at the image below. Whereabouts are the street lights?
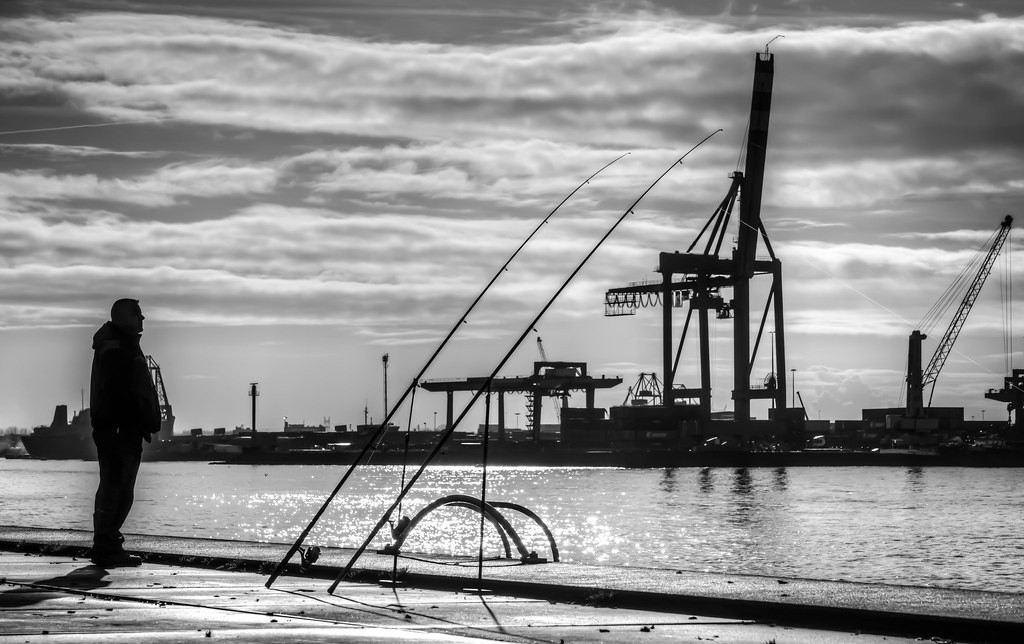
[434,412,437,432]
[791,369,796,408]
[971,416,974,420]
[515,413,520,429]
[981,410,985,420]
[424,422,426,430]
[769,331,777,419]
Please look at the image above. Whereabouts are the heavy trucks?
[812,435,857,448]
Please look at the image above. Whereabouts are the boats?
[5,446,31,459]
[21,405,161,464]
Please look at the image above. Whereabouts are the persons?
[89,298,162,565]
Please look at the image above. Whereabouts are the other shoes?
[92,552,142,567]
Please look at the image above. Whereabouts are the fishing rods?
[260,145,631,591]
[323,123,729,599]
